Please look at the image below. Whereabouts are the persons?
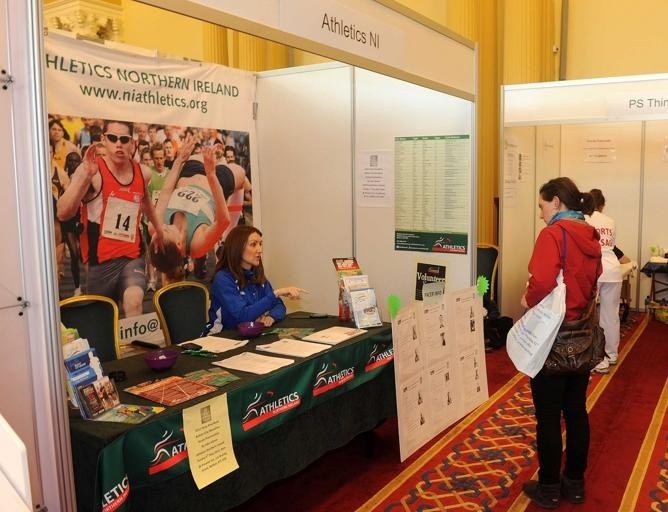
[520,176,606,507]
[48,113,250,318]
[201,225,310,338]
[583,189,625,374]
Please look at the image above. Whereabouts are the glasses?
[103,133,132,143]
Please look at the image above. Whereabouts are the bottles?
[339,291,351,321]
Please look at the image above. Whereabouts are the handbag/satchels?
[506,285,567,379]
[541,289,605,371]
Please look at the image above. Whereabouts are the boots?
[561,468,585,504]
[523,471,562,509]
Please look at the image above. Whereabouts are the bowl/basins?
[237,321,264,338]
[144,349,179,371]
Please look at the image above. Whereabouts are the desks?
[640,259,668,303]
[67,312,398,511]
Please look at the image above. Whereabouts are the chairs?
[476,242,500,309]
[60,295,121,362]
[154,280,210,347]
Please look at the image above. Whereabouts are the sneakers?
[607,353,617,364]
[591,356,609,374]
